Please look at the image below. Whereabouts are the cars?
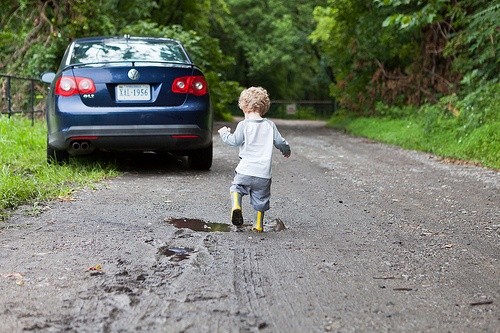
[41,33,215,173]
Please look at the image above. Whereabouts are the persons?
[217,86,291,231]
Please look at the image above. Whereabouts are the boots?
[230,193,244,226]
[251,210,264,233]
